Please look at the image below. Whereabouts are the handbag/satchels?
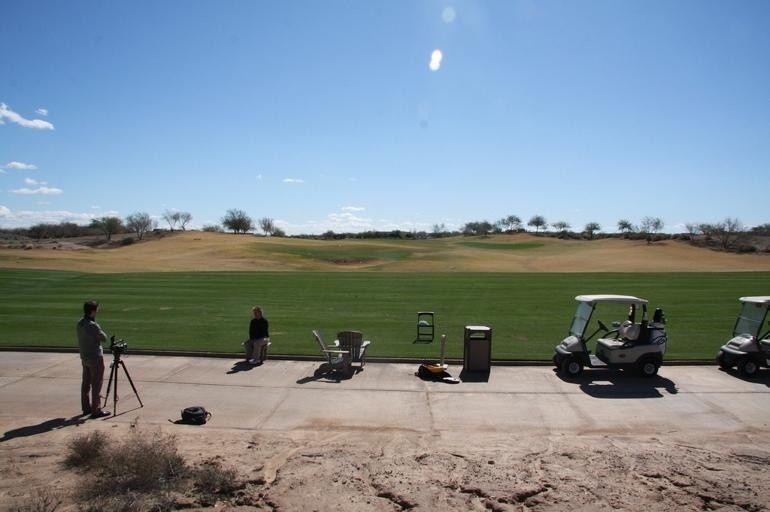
[181,406,211,425]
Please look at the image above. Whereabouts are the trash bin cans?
[463,325,492,372]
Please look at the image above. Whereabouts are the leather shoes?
[83,404,93,415]
[90,411,111,418]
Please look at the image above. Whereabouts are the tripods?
[104,359,143,416]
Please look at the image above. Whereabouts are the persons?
[74,298,112,418]
[244,303,271,365]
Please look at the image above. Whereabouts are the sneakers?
[249,358,257,365]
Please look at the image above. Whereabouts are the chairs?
[597,320,640,348]
[312,329,354,383]
[337,331,370,374]
[417,311,436,342]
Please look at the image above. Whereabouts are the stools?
[241,341,271,363]
